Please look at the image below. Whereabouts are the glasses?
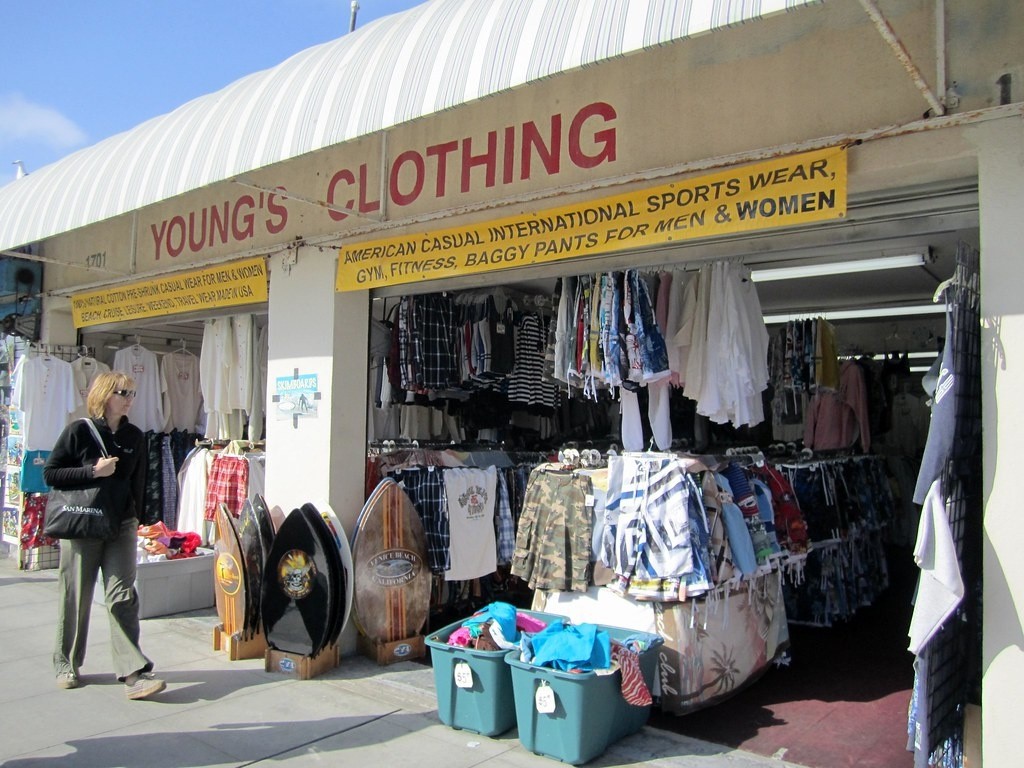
[113,390,136,396]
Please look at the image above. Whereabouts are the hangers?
[174,337,195,361]
[640,256,744,278]
[81,345,96,369]
[131,333,142,350]
[44,344,53,361]
[880,324,918,345]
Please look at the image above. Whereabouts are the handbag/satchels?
[42,418,115,540]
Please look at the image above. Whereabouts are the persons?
[42,369,166,699]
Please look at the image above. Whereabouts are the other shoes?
[57,673,78,689]
[125,676,166,699]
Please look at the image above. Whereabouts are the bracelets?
[92,468,94,477]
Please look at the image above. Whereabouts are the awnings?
[0,0,1024,297]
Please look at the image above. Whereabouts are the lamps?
[743,244,936,283]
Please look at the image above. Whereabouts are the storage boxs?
[93,545,215,621]
[422,600,570,738]
[504,624,665,765]
